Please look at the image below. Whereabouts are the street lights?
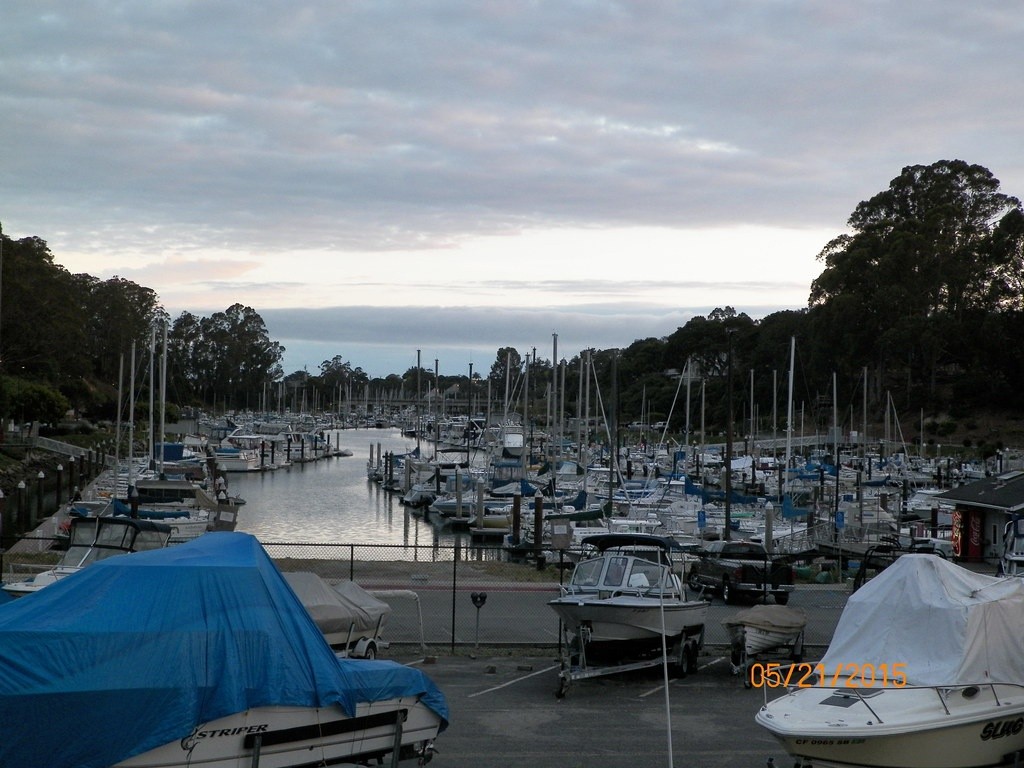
[17,366,26,409]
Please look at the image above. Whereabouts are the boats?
[0,509,393,661]
[719,604,807,655]
[753,553,1024,768]
[0,529,450,768]
[545,532,713,655]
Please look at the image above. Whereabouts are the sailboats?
[66,320,1024,569]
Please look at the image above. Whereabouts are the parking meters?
[469,591,489,651]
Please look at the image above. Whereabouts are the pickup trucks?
[687,540,797,606]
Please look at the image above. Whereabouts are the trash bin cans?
[155,443,184,461]
